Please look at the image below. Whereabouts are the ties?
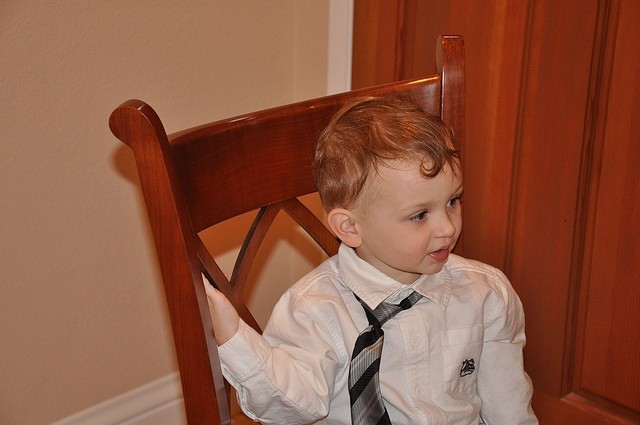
[347,291,424,425]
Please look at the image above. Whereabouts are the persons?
[201,95,541,424]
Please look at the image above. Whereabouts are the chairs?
[109,33,466,425]
